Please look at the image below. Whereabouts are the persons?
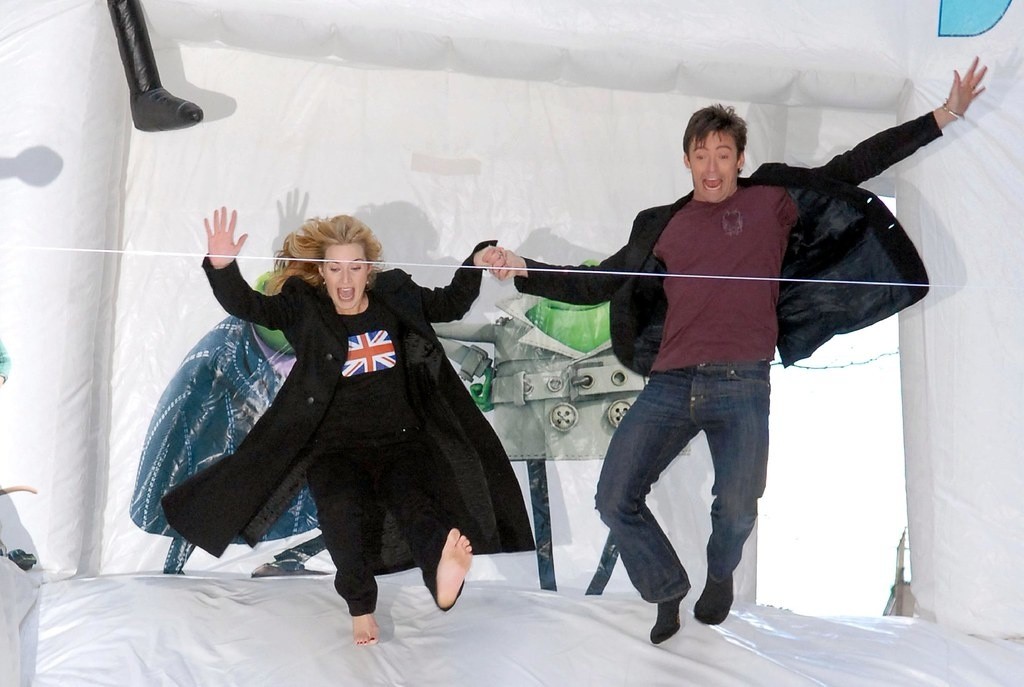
[161,206,537,646]
[486,57,989,643]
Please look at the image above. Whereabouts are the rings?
[971,86,975,89]
[498,252,503,257]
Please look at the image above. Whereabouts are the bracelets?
[943,104,964,119]
